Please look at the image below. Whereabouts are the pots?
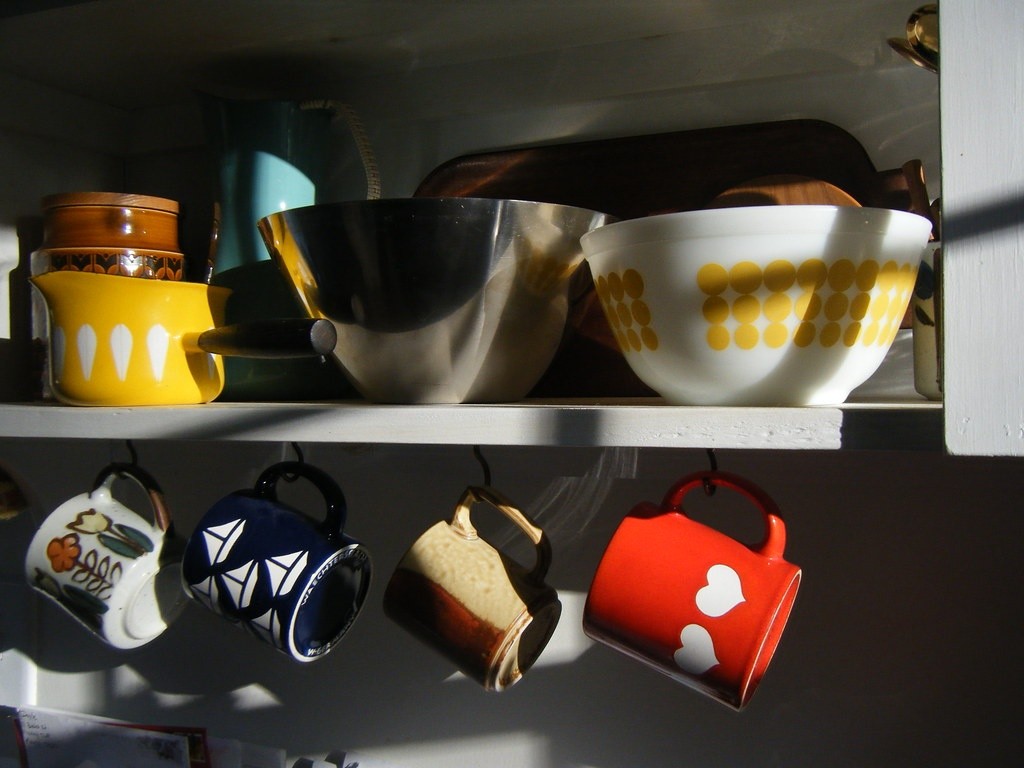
[33,271,337,407]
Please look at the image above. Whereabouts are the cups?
[581,475,806,712]
[24,462,188,647]
[179,460,375,664]
[381,487,563,692]
[913,241,944,402]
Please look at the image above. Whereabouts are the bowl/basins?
[257,198,608,404]
[578,205,933,406]
[43,190,180,251]
[36,246,185,280]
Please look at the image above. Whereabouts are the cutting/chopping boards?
[411,117,916,328]
